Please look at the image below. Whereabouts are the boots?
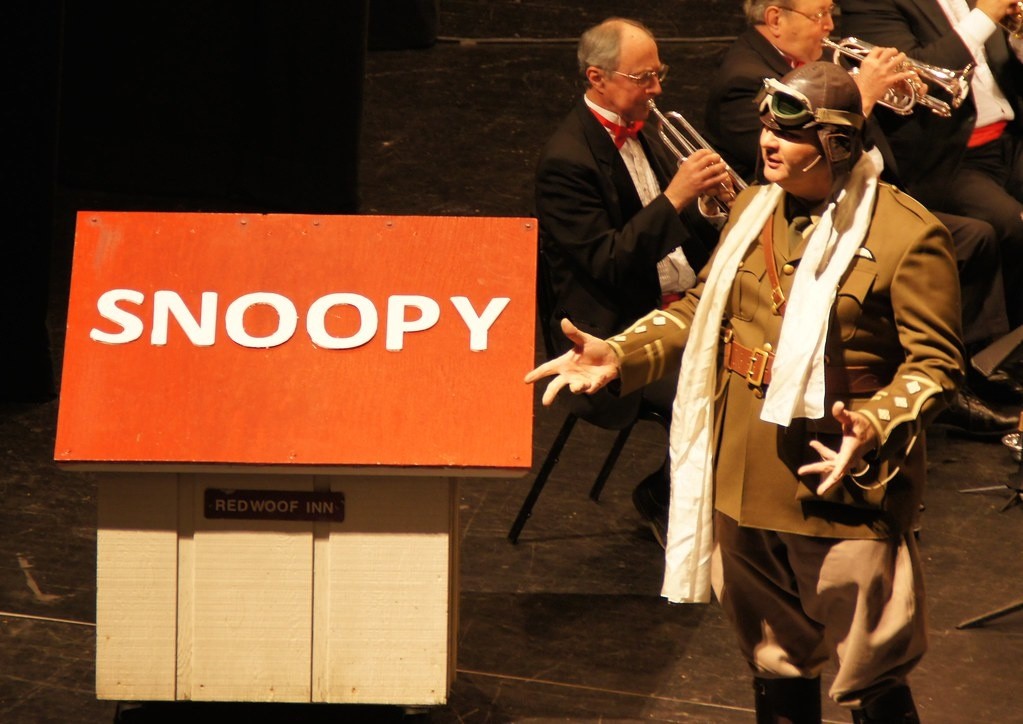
[753,674,822,724]
[850,685,921,724]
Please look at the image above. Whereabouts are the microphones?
[803,154,824,172]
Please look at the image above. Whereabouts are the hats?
[760,61,863,180]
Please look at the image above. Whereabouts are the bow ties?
[589,107,644,148]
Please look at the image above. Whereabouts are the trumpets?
[644,95,749,214]
[821,35,974,118]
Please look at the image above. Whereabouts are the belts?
[718,327,899,395]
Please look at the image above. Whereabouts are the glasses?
[613,64,668,83]
[758,78,863,129]
[779,4,835,23]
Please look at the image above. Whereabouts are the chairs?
[507,233,673,543]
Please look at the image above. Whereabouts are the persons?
[525,61,965,724]
[532,17,737,548]
[706,0,1022,434]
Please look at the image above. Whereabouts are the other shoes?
[970,370,1023,400]
[932,386,1018,435]
[632,457,671,549]
